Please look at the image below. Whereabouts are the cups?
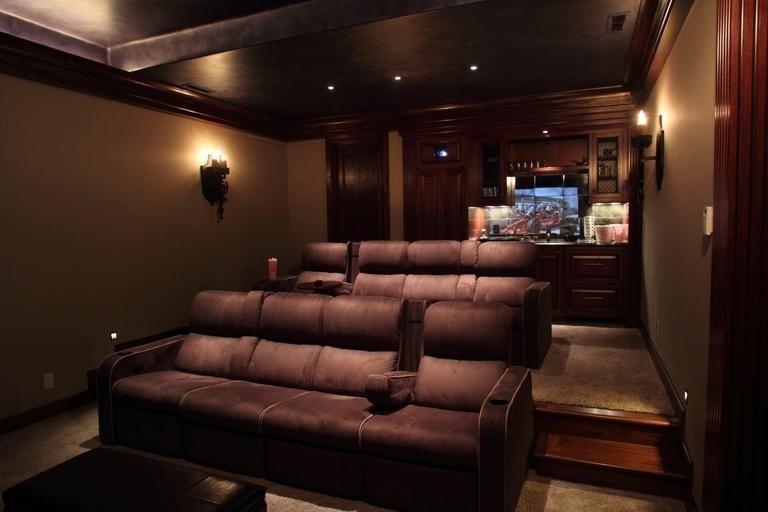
[509,160,545,172]
[592,225,612,242]
[546,227,552,241]
[583,215,595,240]
[268,260,278,279]
[611,223,626,243]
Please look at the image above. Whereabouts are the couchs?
[292,238,552,368]
[95,285,535,507]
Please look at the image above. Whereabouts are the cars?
[484,200,581,236]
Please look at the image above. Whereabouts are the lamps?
[199,149,230,221]
[631,108,666,194]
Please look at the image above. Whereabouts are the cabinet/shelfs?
[553,246,642,322]
[496,128,589,190]
[592,130,625,200]
